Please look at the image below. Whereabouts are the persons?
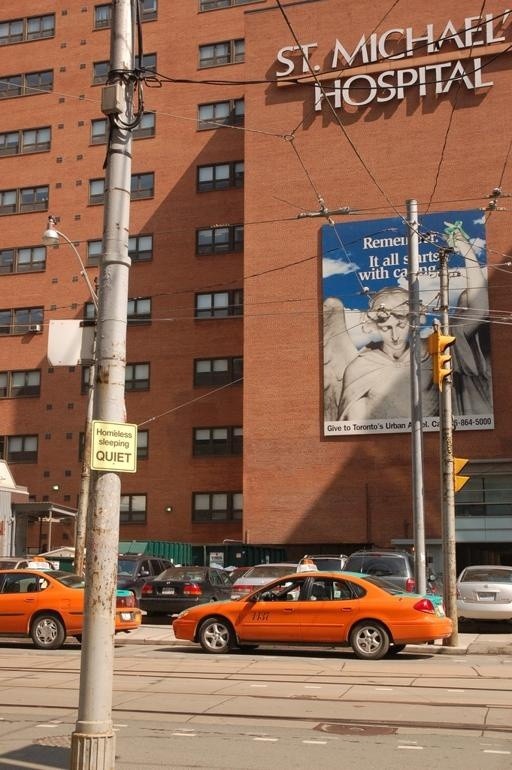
[338,222,489,421]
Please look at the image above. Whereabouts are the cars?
[170,557,455,661]
[455,565,512,632]
[0,556,143,650]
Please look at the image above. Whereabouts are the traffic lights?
[439,328,456,393]
[454,459,470,495]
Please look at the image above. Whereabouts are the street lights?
[40,214,102,587]
